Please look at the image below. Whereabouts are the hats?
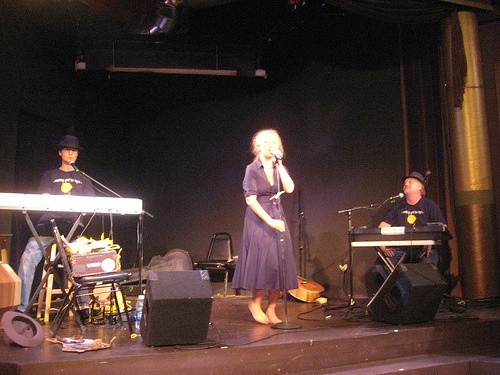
[400,171,428,186]
[56,135,84,151]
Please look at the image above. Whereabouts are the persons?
[16,135,96,313]
[231,130,299,324]
[365,172,447,302]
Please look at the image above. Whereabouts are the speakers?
[140,269,214,347]
[367,262,449,325]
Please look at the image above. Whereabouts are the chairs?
[193,232,240,300]
[49,226,134,338]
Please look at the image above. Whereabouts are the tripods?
[320,198,393,312]
[77,170,154,318]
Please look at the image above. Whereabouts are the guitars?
[287,276,325,303]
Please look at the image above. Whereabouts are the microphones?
[390,192,404,200]
[270,150,282,159]
[67,159,79,172]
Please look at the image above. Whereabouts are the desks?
[350,240,443,316]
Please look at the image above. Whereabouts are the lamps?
[146,4,175,35]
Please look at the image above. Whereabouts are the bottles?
[135,294,144,329]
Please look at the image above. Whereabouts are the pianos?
[0,193,144,335]
[347,224,455,311]
